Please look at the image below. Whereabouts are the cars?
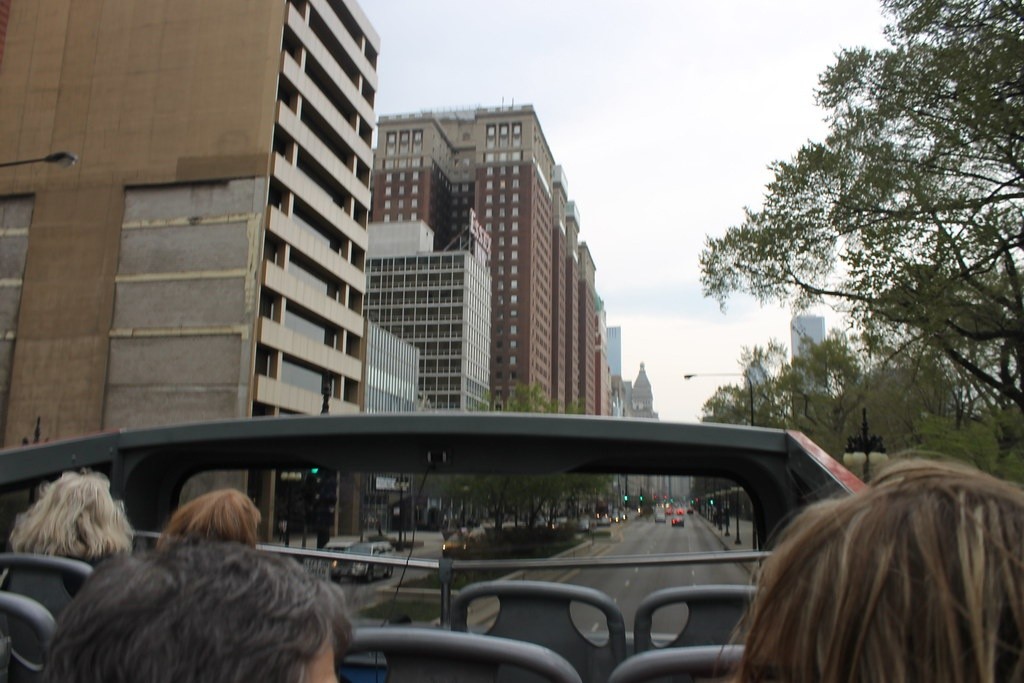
[655,513,666,522]
[672,517,684,527]
[611,512,625,523]
[330,542,397,584]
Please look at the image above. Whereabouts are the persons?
[737,459,1024,683]
[43,540,353,683]
[157,489,261,551]
[3,473,132,619]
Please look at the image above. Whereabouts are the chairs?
[347,626,584,683]
[0,591,55,683]
[451,580,628,683]
[608,644,746,683]
[634,584,754,652]
[0,552,94,618]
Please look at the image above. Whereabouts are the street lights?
[842,407,890,485]
[0,151,77,167]
[684,373,757,551]
[394,473,410,552]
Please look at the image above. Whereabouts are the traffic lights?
[311,468,320,476]
